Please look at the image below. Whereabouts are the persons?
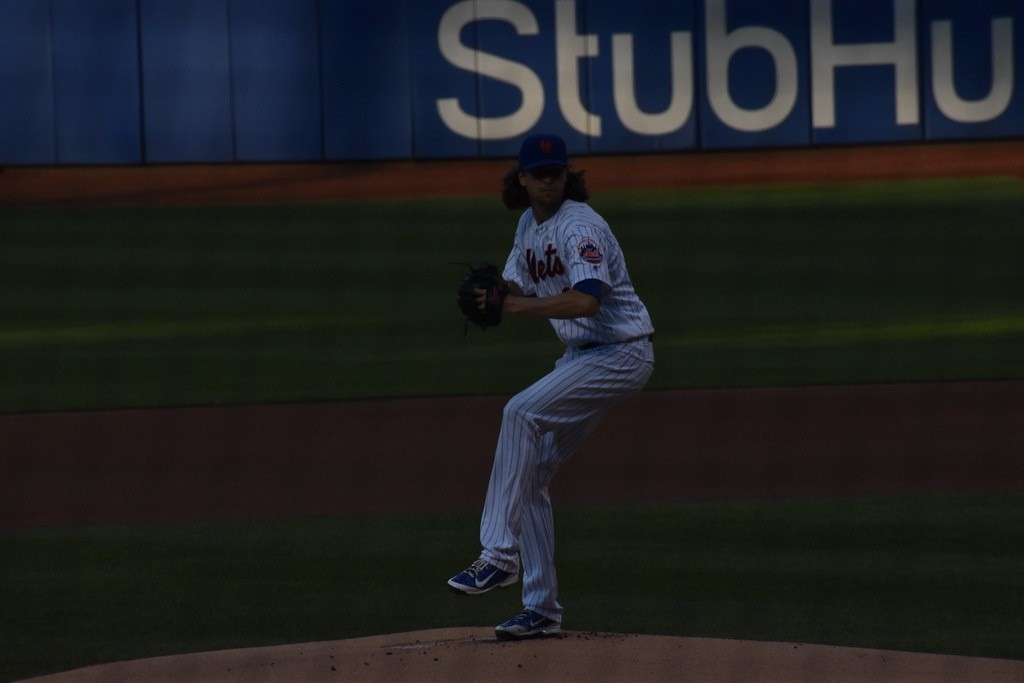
[448,134,655,638]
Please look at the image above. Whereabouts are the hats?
[520,134,567,169]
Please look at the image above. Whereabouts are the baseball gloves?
[456,264,511,328]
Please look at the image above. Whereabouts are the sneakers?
[447,558,519,595]
[494,609,562,640]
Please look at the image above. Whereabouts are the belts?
[578,335,653,351]
